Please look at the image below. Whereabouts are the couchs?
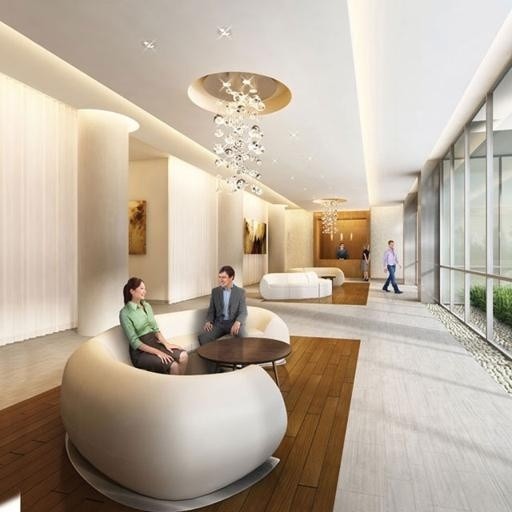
[61,306,291,512]
[258,266,345,302]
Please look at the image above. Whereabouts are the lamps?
[209,70,265,195]
